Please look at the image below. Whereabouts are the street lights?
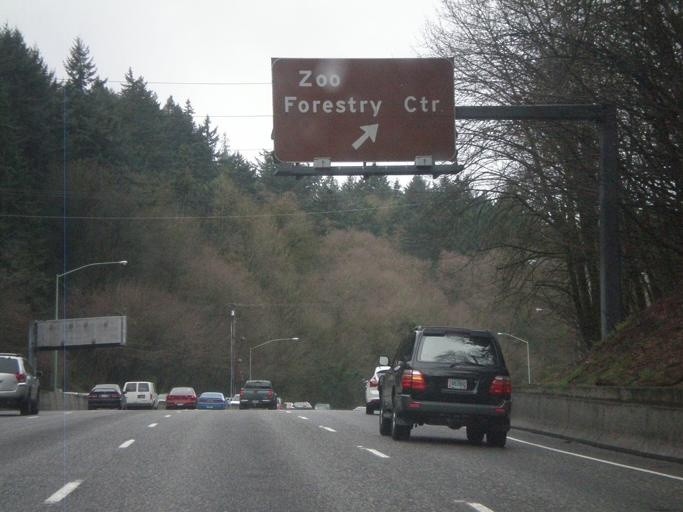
[228,310,235,394]
[248,337,302,380]
[53,261,129,391]
[495,331,531,383]
[533,306,551,314]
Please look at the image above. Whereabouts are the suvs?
[363,365,391,414]
[376,325,513,446]
[0,352,42,416]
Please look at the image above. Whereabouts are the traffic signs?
[271,58,456,162]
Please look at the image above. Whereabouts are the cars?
[86,378,331,410]
[352,406,366,412]
